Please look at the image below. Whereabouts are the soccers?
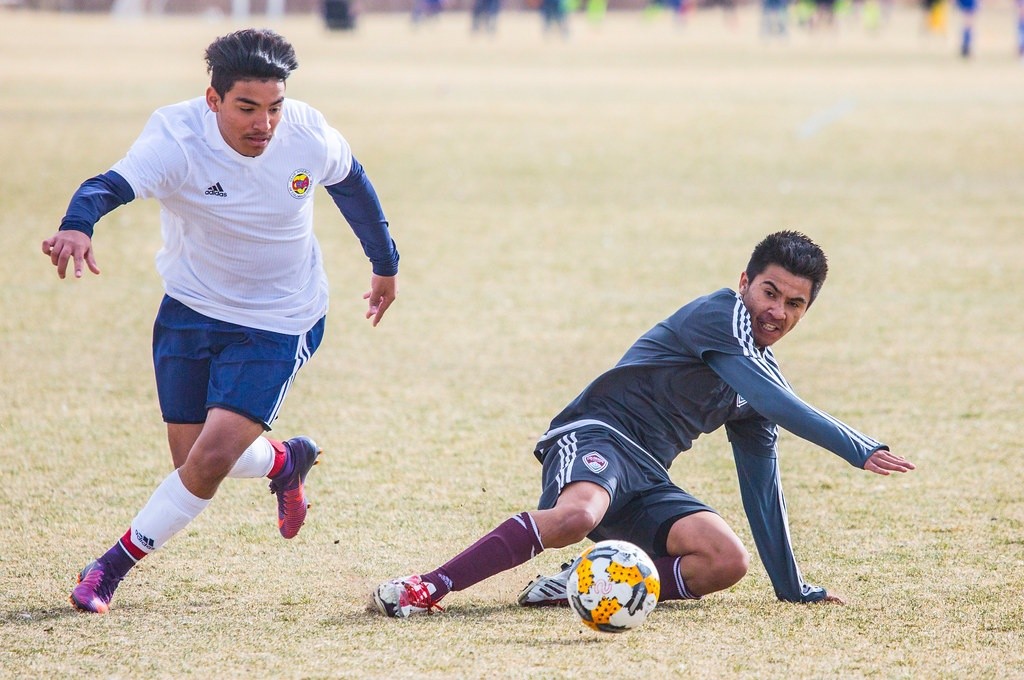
[566,540,661,634]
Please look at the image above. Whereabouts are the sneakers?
[70,559,125,613]
[268,435,322,539]
[374,574,445,617]
[518,559,575,609]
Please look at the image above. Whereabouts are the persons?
[324,1,1024,70]
[39,29,400,615]
[366,230,916,616]
